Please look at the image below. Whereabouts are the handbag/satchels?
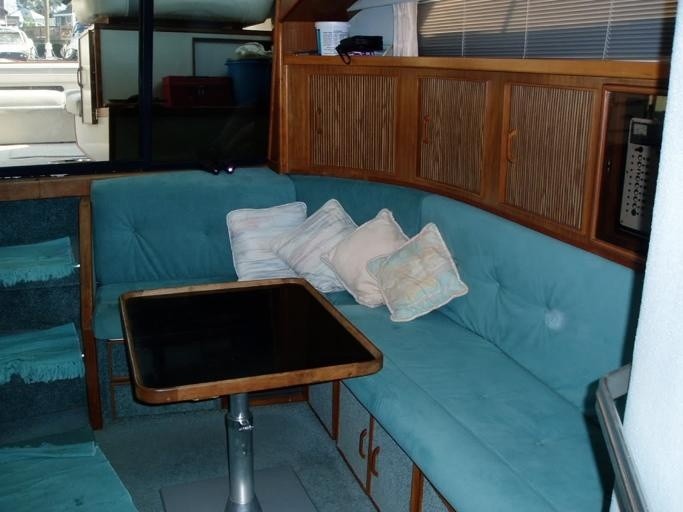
[340,35,383,51]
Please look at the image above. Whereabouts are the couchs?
[86,166,646,511]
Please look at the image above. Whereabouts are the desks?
[118,277,385,510]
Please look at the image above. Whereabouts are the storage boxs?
[162,77,232,109]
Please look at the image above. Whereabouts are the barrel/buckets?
[314,21,352,57]
[224,56,273,110]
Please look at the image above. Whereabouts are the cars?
[0,25,36,61]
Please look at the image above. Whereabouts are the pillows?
[224,197,469,323]
